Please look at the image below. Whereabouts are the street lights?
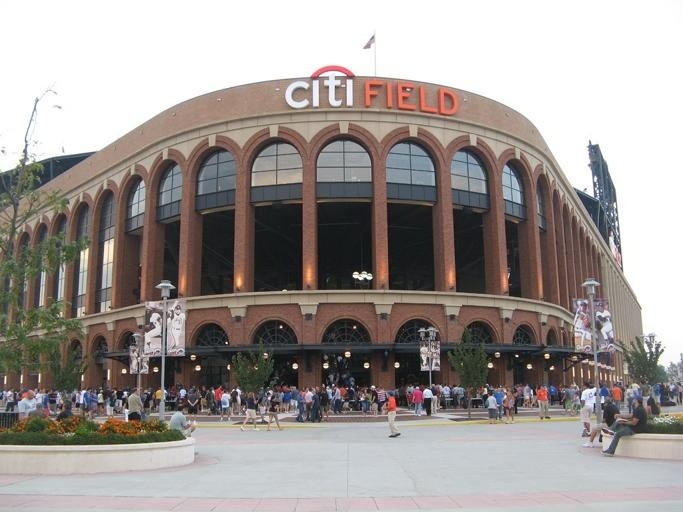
[581,280,601,437]
[131,332,143,394]
[157,280,176,419]
[417,326,440,388]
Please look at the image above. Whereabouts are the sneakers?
[582,441,594,448]
[600,451,614,457]
[389,433,400,437]
[582,428,591,436]
[489,416,552,423]
[414,411,438,416]
[240,426,284,432]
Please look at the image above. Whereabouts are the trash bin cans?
[0,412,19,429]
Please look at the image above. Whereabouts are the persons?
[168,404,196,437]
[431,347,440,366]
[420,343,428,366]
[601,395,648,455]
[140,349,148,372]
[595,302,614,343]
[130,349,137,372]
[579,381,594,437]
[583,400,620,446]
[574,301,589,329]
[384,391,401,437]
[167,305,184,348]
[144,310,162,348]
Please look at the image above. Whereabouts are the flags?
[363,35,375,49]
